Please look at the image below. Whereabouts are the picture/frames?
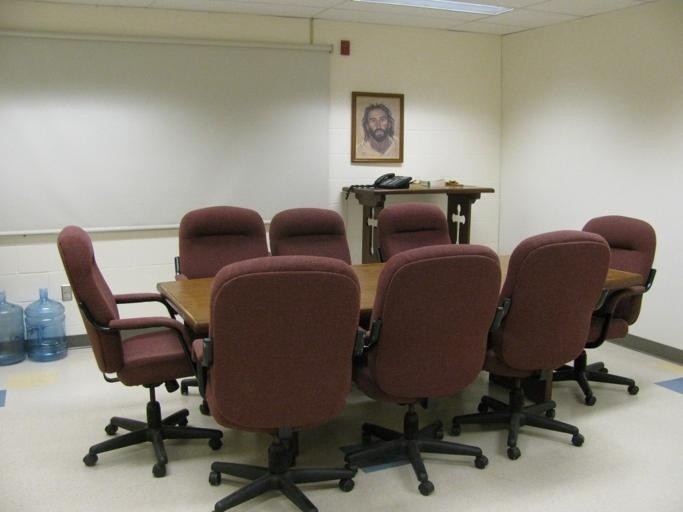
[351,90,405,162]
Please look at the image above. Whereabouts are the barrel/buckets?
[0,289,23,364]
[25,285,67,362]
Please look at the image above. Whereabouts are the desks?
[343,183,495,263]
[155,255,644,405]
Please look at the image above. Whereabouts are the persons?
[355,103,399,159]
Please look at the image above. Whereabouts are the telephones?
[375,172,412,189]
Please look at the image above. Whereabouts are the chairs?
[176,206,274,416]
[552,216,656,406]
[192,254,361,512]
[57,224,224,478]
[344,244,502,495]
[378,203,452,261]
[451,231,611,461]
[269,206,353,264]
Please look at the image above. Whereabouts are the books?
[416,177,446,186]
